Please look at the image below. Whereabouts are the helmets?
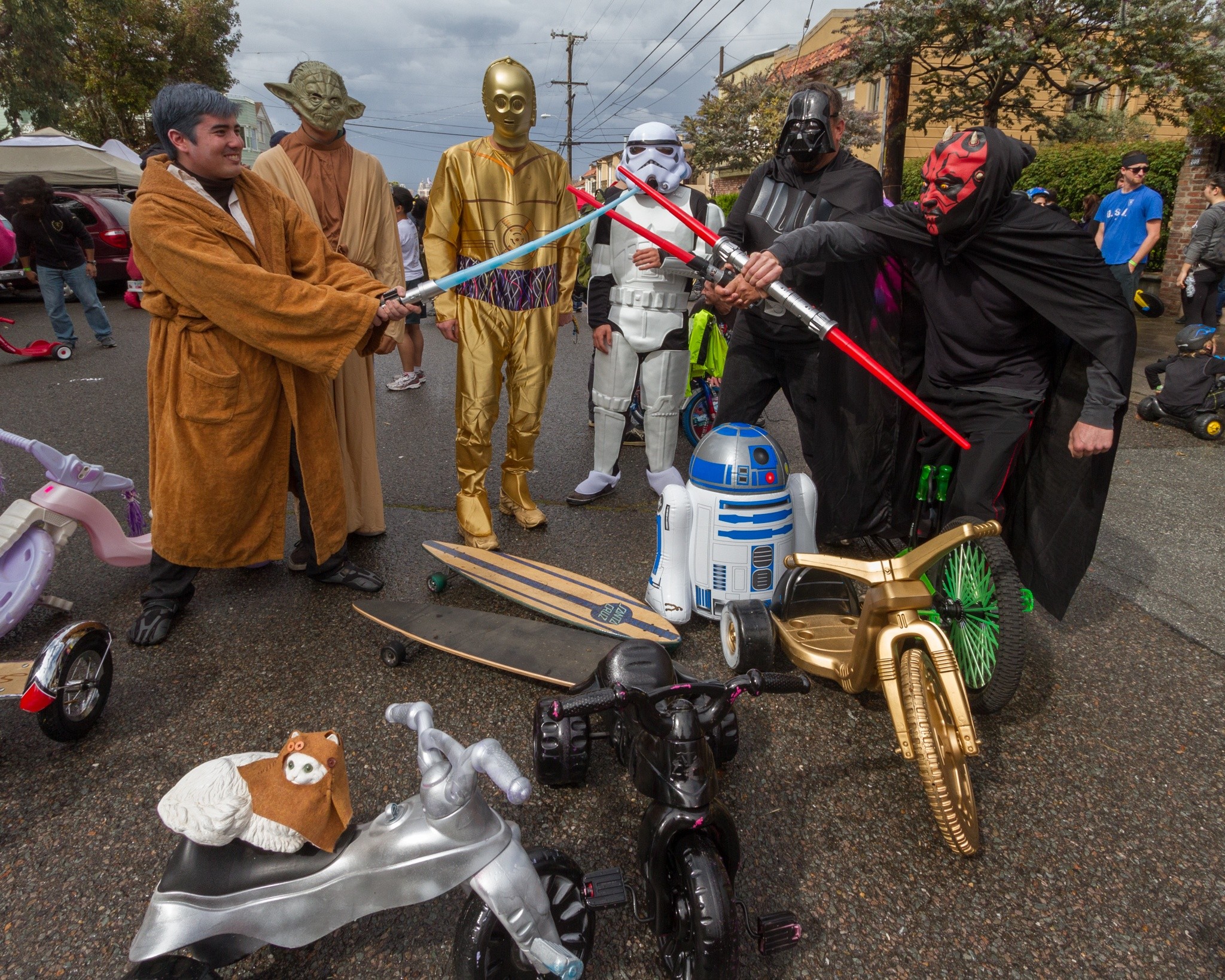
[482,58,537,148]
[1026,187,1049,199]
[1175,324,1216,351]
[615,121,692,194]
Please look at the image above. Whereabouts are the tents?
[0,127,143,195]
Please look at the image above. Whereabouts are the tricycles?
[719,518,1008,857]
[526,637,815,980]
[122,700,597,980]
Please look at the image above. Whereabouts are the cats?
[157,731,340,855]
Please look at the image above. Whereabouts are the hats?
[269,130,291,148]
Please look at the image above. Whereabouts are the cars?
[52,188,135,303]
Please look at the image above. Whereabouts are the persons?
[689,279,739,387]
[1144,324,1225,418]
[128,83,422,646]
[1045,189,1068,217]
[421,55,581,551]
[741,127,1137,622]
[1025,187,1050,206]
[566,120,709,505]
[386,187,428,391]
[712,87,883,545]
[1093,150,1163,309]
[1079,194,1099,231]
[4,173,118,348]
[1087,172,1124,238]
[1175,171,1225,327]
[251,61,406,575]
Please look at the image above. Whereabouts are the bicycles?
[630,314,721,447]
[0,430,278,638]
[895,465,1035,715]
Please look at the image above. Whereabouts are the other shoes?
[622,426,646,446]
[645,462,685,496]
[588,419,595,427]
[573,307,582,313]
[566,469,621,505]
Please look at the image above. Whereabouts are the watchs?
[88,260,96,265]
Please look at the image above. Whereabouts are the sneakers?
[458,519,500,551]
[288,538,315,570]
[61,342,75,349]
[385,372,421,391]
[756,414,765,428]
[499,486,547,528]
[127,606,175,647]
[392,370,426,383]
[102,337,117,348]
[309,557,385,592]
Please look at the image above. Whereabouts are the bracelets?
[23,268,31,271]
[1129,260,1137,266]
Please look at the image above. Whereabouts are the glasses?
[1125,166,1150,174]
